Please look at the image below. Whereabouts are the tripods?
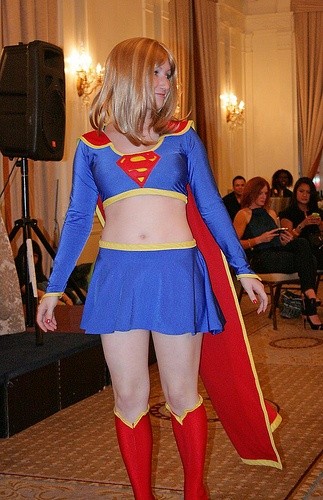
[7,156,86,347]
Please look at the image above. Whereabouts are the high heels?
[302,310,323,330]
[304,293,321,306]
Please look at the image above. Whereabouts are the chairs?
[232,193,323,332]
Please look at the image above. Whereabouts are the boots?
[165,394,209,500]
[113,404,155,500]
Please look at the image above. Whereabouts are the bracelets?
[248,239,252,248]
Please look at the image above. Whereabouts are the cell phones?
[312,212,319,217]
[275,227,289,235]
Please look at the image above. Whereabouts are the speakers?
[1,39,66,161]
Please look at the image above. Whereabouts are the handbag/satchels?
[280,290,303,319]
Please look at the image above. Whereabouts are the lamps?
[75,62,105,104]
[220,91,245,126]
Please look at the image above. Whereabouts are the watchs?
[298,225,303,229]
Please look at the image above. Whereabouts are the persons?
[13,239,86,306]
[35,36,283,500]
[221,176,246,222]
[269,169,293,197]
[278,177,323,269]
[231,177,323,331]
